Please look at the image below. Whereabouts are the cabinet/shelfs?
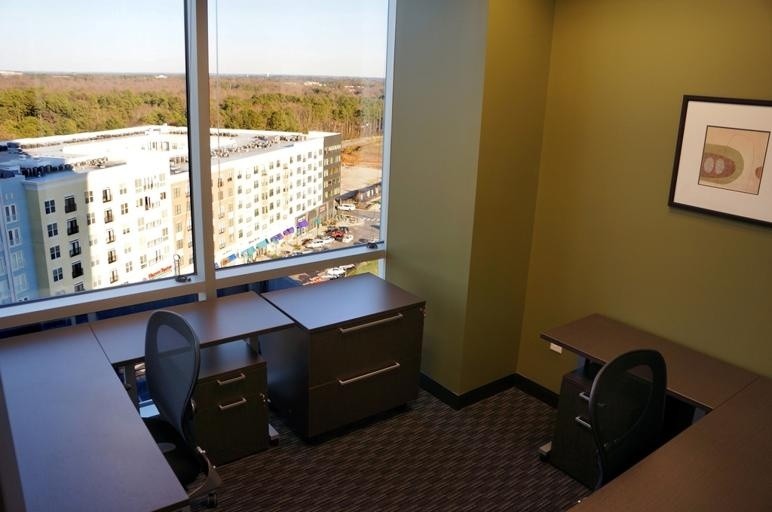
[181,339,271,467]
[262,269,427,441]
[548,361,697,491]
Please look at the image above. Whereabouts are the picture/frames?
[669,93,772,228]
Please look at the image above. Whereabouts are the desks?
[0,322,192,511]
[85,289,295,416]
[538,375,771,511]
[536,312,761,461]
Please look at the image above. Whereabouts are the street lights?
[173,254,181,276]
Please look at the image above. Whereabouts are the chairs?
[139,307,221,511]
[590,348,669,490]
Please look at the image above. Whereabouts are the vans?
[336,203,356,212]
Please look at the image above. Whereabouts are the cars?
[285,227,356,282]
